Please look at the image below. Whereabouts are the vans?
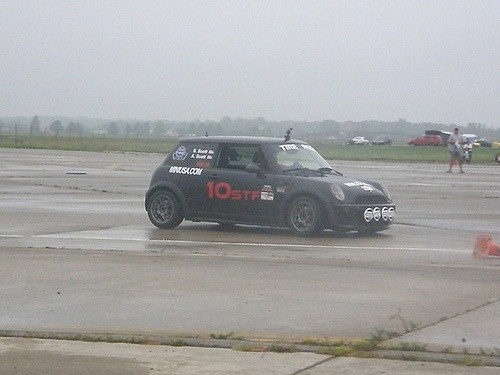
[408,134,442,146]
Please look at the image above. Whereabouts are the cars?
[145,128,399,235]
[352,136,369,144]
[372,136,394,146]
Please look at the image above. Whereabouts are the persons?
[462,141,473,160]
[444,128,466,172]
